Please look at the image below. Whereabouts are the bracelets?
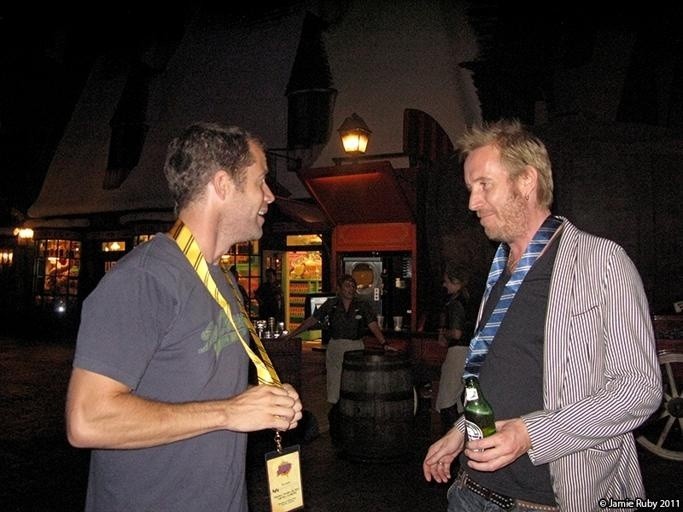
[382,342,389,347]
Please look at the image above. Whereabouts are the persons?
[64,123,304,512]
[230,266,251,305]
[435,268,478,430]
[423,121,662,512]
[254,269,283,323]
[279,274,398,405]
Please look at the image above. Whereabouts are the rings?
[438,463,444,464]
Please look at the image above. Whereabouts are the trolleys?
[633,300,683,461]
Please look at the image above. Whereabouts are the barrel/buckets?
[251,338,302,393]
[338,349,408,456]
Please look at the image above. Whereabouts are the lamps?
[336,112,373,165]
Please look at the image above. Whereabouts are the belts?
[457,469,560,511]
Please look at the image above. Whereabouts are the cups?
[376,315,384,331]
[391,314,403,331]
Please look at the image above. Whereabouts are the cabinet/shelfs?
[288,278,322,341]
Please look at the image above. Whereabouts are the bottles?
[462,373,496,452]
[253,315,292,340]
